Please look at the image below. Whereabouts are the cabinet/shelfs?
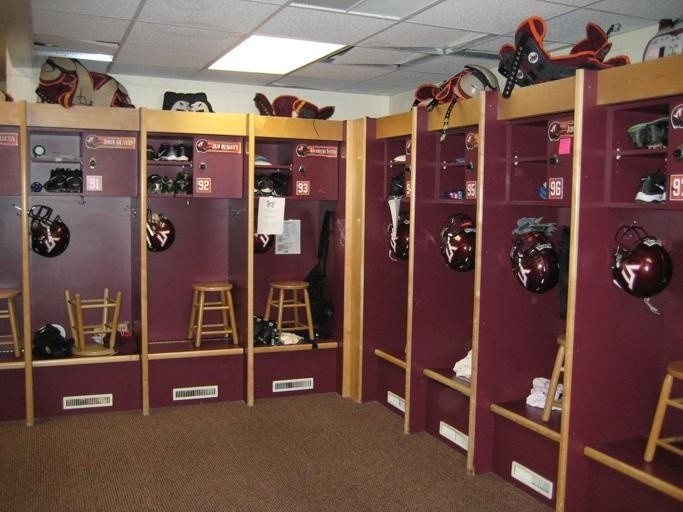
[423,375,469,455]
[2,369,25,422]
[602,94,683,209]
[0,99,352,426]
[33,359,141,421]
[435,133,477,206]
[148,353,246,410]
[256,136,339,200]
[353,56,683,510]
[384,140,410,202]
[495,411,559,509]
[24,125,139,198]
[377,356,405,420]
[254,347,338,400]
[1,124,21,196]
[147,131,243,199]
[507,113,573,206]
[591,458,683,511]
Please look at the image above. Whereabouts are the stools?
[188,280,240,349]
[542,333,565,423]
[645,358,682,463]
[62,287,125,356]
[1,287,22,357]
[266,279,314,343]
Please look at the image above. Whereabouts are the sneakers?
[146,142,193,198]
[633,171,667,203]
[626,114,671,152]
[255,174,288,199]
[253,315,280,348]
[41,164,84,194]
[152,140,189,164]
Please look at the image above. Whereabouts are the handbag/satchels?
[302,264,337,326]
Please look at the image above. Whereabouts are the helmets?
[388,211,412,262]
[504,218,561,292]
[146,218,176,253]
[31,218,71,258]
[254,233,273,255]
[612,236,674,299]
[437,215,477,272]
